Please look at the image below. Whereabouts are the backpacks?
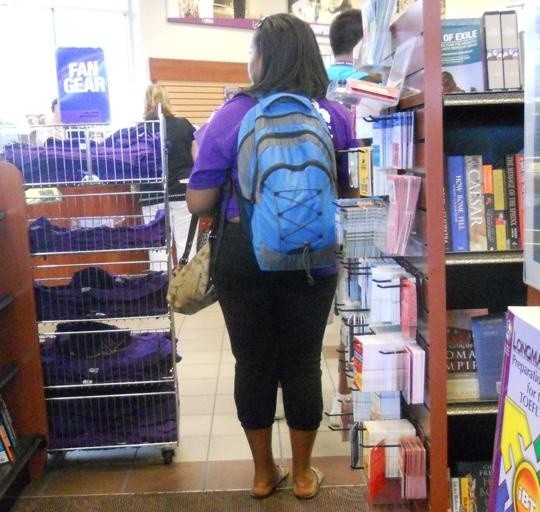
[236,92,339,283]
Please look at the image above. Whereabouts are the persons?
[37,99,66,146]
[185,13,365,499]
[138,85,198,275]
[325,9,377,427]
[442,71,465,94]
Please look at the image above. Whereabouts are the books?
[446,460,492,511]
[487,305,540,511]
[0,398,17,464]
[441,11,521,92]
[444,154,523,251]
[446,309,507,402]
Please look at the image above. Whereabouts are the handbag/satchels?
[166,239,218,315]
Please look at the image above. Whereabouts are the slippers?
[250,465,324,499]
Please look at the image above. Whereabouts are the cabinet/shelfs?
[390,0,540,512]
[0,104,179,509]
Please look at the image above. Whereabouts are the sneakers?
[329,411,342,427]
